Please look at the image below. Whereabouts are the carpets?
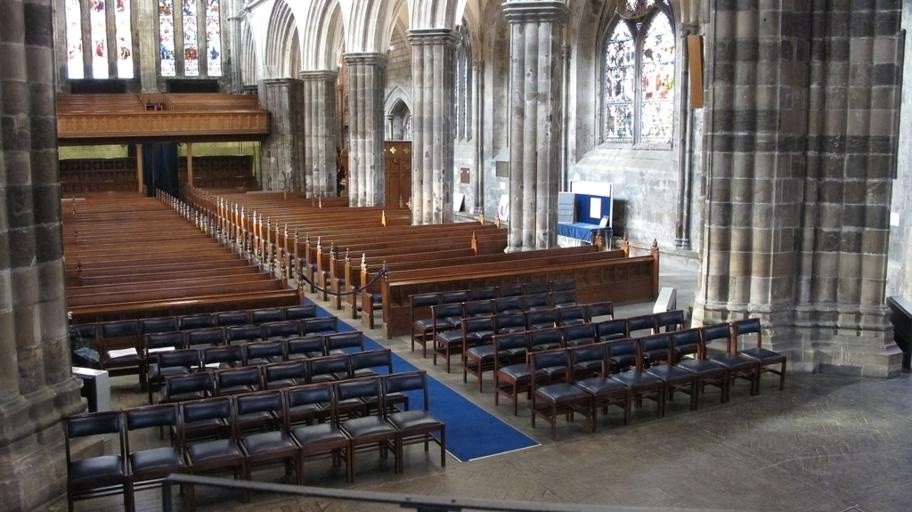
[262,271,543,464]
